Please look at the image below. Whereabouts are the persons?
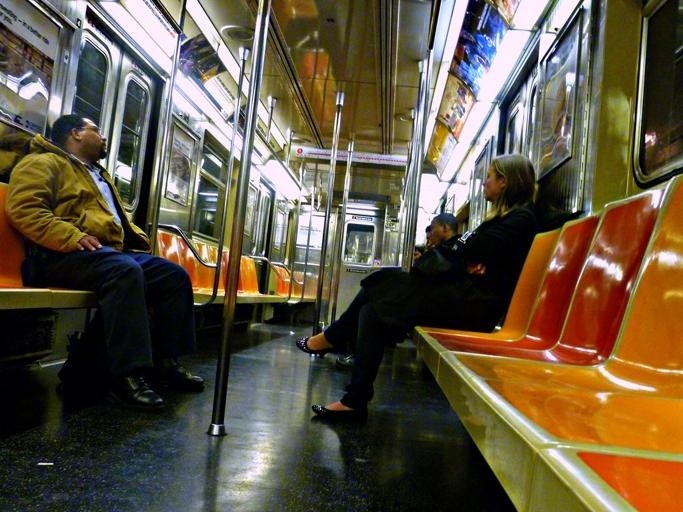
[3,113,206,413]
[424,226,436,249]
[288,153,536,421]
[414,245,424,263]
[334,214,464,367]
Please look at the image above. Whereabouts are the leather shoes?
[311,403,371,419]
[295,337,350,355]
[107,375,165,412]
[159,365,209,390]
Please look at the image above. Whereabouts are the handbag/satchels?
[412,237,470,280]
